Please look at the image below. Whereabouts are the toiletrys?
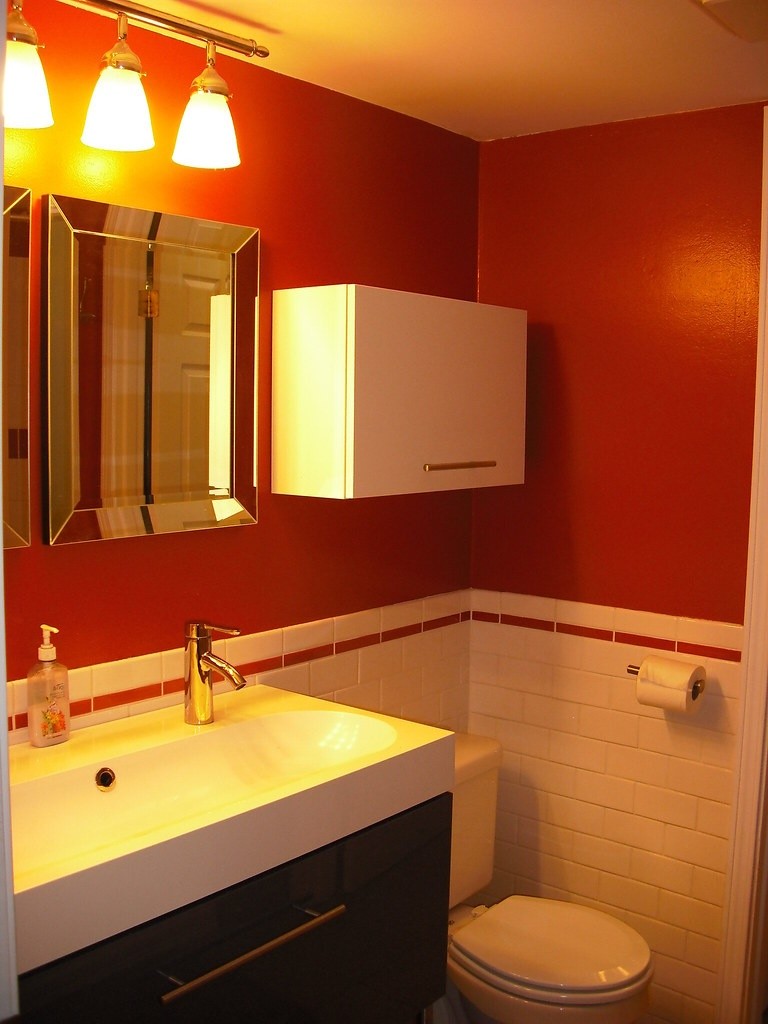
[26,624,71,748]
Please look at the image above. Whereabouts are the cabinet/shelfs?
[17,789,453,1024]
[271,284,527,500]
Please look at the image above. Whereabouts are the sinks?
[8,712,397,880]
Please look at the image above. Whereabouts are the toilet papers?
[636,654,707,715]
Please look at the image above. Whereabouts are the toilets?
[431,733,655,1024]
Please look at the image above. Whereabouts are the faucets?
[183,619,247,726]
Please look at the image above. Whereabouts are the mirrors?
[2,194,258,547]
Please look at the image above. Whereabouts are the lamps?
[4,0,268,170]
